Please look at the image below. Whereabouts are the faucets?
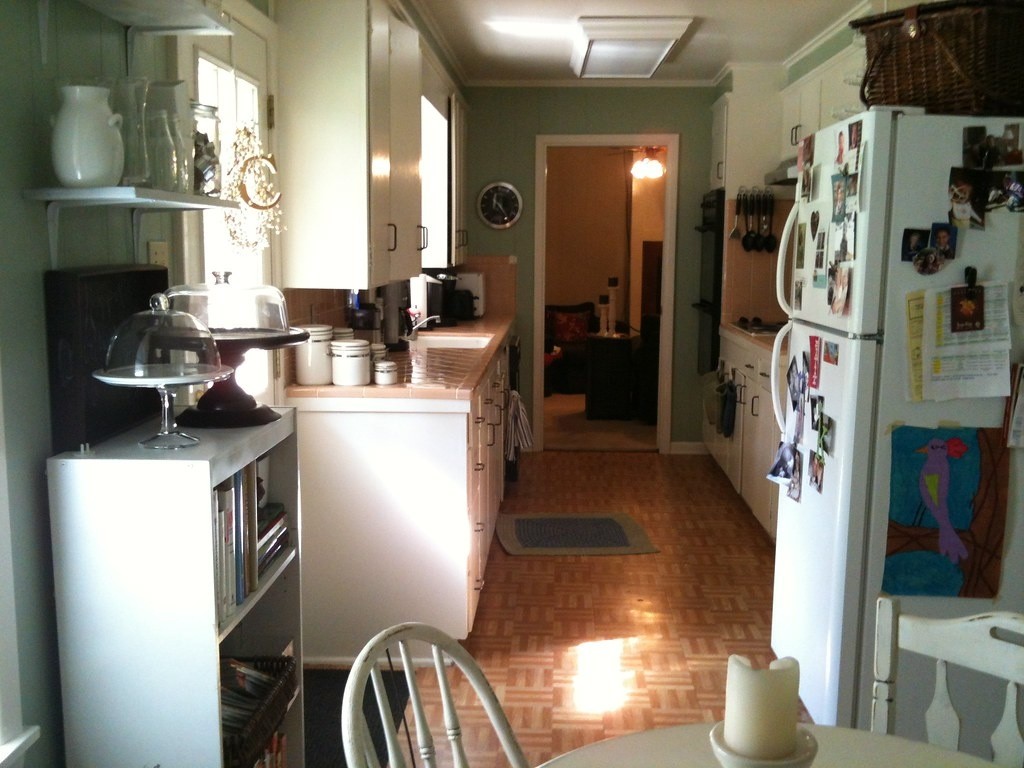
[407,313,441,341]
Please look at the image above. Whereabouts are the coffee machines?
[428,268,464,327]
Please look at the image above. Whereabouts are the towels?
[504,390,536,464]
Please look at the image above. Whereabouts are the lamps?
[569,18,693,83]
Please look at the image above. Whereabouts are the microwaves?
[455,272,487,317]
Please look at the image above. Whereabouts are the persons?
[903,232,923,261]
[789,118,865,316]
[1003,175,1023,193]
[934,226,953,259]
[982,134,1002,172]
[766,335,844,503]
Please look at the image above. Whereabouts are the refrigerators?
[770,106,1024,756]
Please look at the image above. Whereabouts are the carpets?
[495,507,661,558]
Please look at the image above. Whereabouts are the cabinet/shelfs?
[706,336,787,539]
[421,85,472,269]
[709,105,732,193]
[296,335,511,667]
[46,406,309,767]
[274,0,427,289]
[780,43,866,162]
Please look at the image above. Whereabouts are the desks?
[587,335,635,420]
[534,725,999,767]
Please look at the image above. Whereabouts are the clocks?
[477,181,523,231]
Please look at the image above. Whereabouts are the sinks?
[408,336,491,348]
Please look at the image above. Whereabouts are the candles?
[726,652,804,758]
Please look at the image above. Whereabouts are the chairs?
[546,303,596,368]
[867,595,1024,768]
[341,624,528,768]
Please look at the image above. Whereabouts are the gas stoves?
[729,316,788,337]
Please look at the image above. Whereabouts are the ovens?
[691,187,723,374]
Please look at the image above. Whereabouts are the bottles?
[296,307,398,385]
[51,75,223,197]
